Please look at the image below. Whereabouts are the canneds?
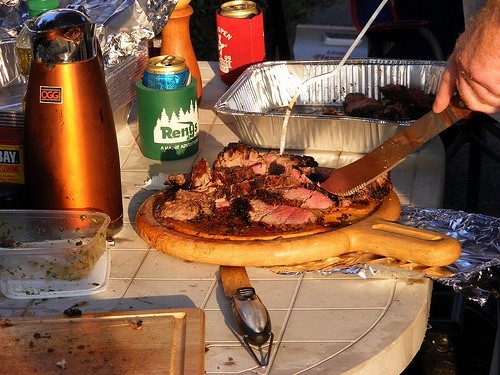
[142,54,191,90]
[220,0,258,19]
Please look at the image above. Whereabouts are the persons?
[433,0,500,115]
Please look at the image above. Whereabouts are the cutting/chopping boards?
[0,307,207,375]
[131,166,463,269]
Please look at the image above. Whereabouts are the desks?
[0,60,445,375]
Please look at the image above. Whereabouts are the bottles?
[159,0,203,105]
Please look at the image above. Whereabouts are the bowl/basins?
[0,209,112,297]
[213,55,449,153]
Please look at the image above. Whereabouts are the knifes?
[216,264,274,367]
[322,101,472,195]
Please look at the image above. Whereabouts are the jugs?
[18,2,123,238]
